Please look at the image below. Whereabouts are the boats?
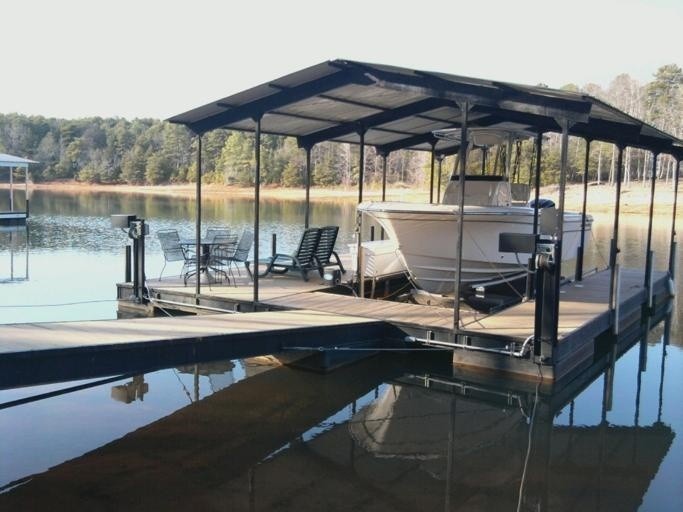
[347,383,524,493]
[356,127,593,294]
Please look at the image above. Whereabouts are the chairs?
[244,226,347,283]
[155,225,255,291]
